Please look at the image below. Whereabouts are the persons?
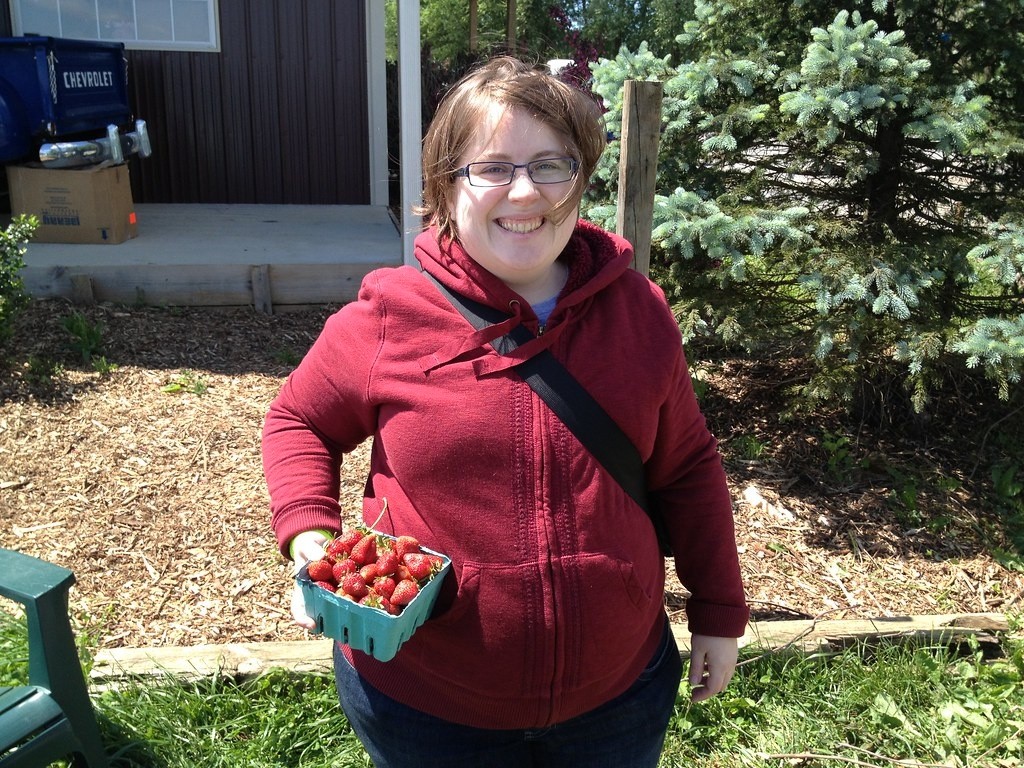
[259,56,751,768]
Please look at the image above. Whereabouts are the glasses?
[448,157,577,187]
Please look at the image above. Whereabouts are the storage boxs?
[0,32,133,144]
[296,524,452,660]
[6,159,139,244]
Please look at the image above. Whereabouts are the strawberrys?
[306,526,444,616]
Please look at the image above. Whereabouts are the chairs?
[0,547,109,768]
